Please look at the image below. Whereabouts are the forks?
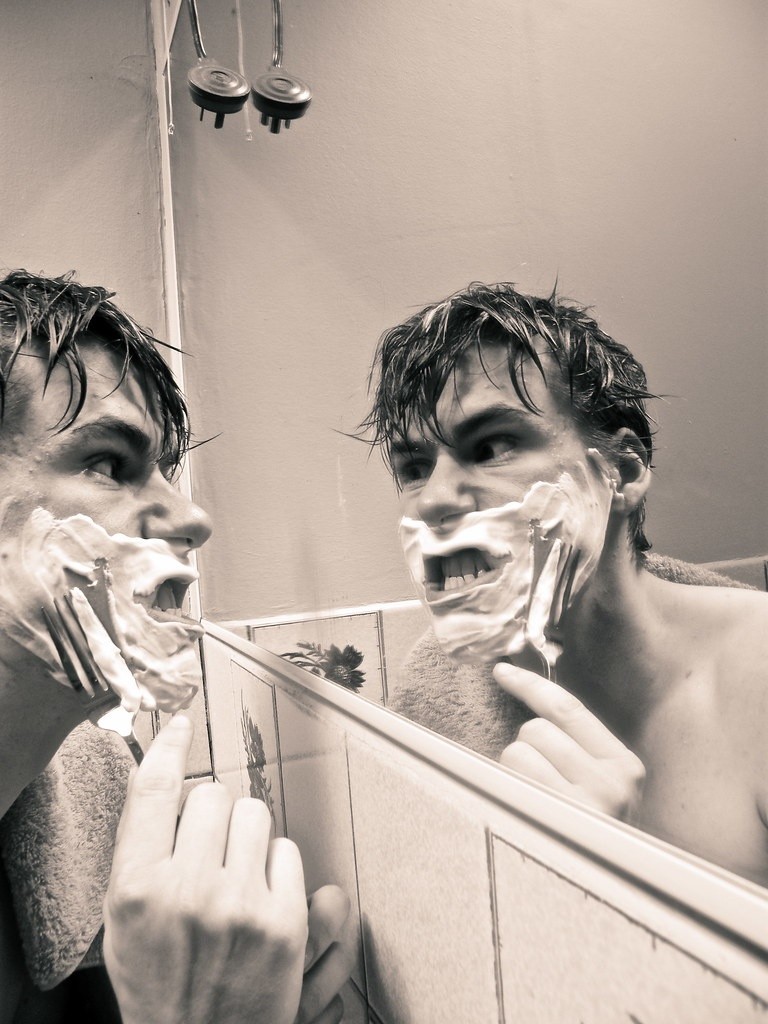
[40,588,146,768]
[521,537,584,685]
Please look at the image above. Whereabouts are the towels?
[386,553,758,763]
[7,719,137,994]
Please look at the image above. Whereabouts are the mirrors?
[162,0,768,959]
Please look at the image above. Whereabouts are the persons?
[0,270,356,1024]
[372,271,766,887]
